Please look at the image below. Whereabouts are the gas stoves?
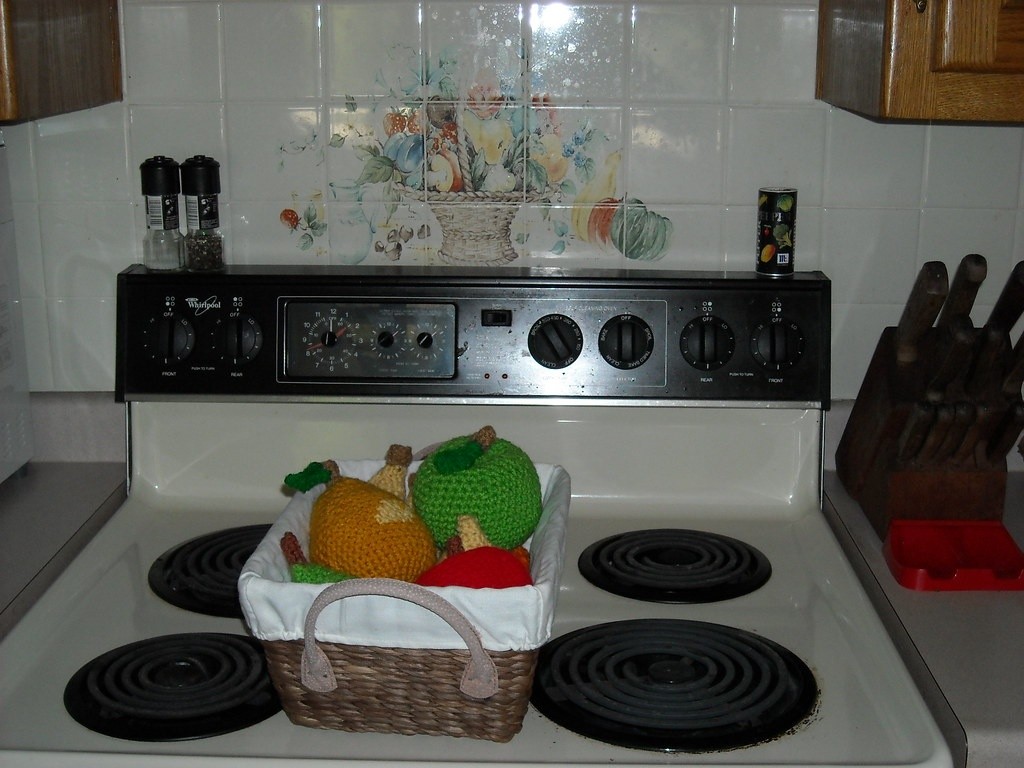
[0,266,954,768]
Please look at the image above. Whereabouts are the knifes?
[893,252,1023,413]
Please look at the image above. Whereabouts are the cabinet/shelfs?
[817,0,1024,128]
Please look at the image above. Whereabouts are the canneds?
[754,186,799,276]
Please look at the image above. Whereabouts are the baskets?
[238,443,573,744]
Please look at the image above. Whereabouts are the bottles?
[139,155,188,273]
[180,155,225,273]
